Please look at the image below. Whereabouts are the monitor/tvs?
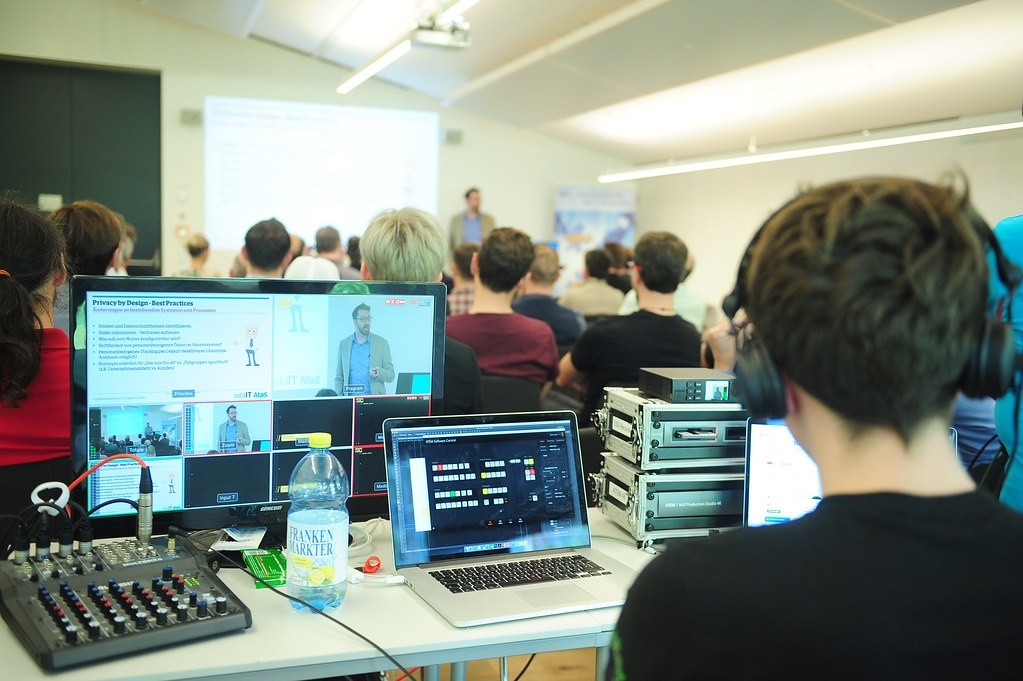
[66,275,447,563]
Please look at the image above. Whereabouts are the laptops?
[743,417,822,529]
[382,410,639,628]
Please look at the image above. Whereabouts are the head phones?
[720,194,1023,421]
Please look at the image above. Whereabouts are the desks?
[0,502,658,681]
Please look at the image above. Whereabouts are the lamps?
[596,107,1023,183]
[336,0,480,95]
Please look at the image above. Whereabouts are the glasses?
[230,411,237,414]
[356,316,372,321]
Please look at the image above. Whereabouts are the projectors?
[409,28,472,51]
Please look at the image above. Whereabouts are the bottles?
[287,432,350,614]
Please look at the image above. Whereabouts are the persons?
[177,235,221,277]
[556,250,625,316]
[335,303,396,396]
[49,200,136,335]
[556,231,715,430]
[97,422,169,456]
[511,243,587,345]
[0,200,70,467]
[359,206,483,416]
[605,243,633,295]
[447,227,560,383]
[446,242,476,315]
[605,177,1023,681]
[231,217,362,280]
[448,188,494,250]
[218,405,251,453]
